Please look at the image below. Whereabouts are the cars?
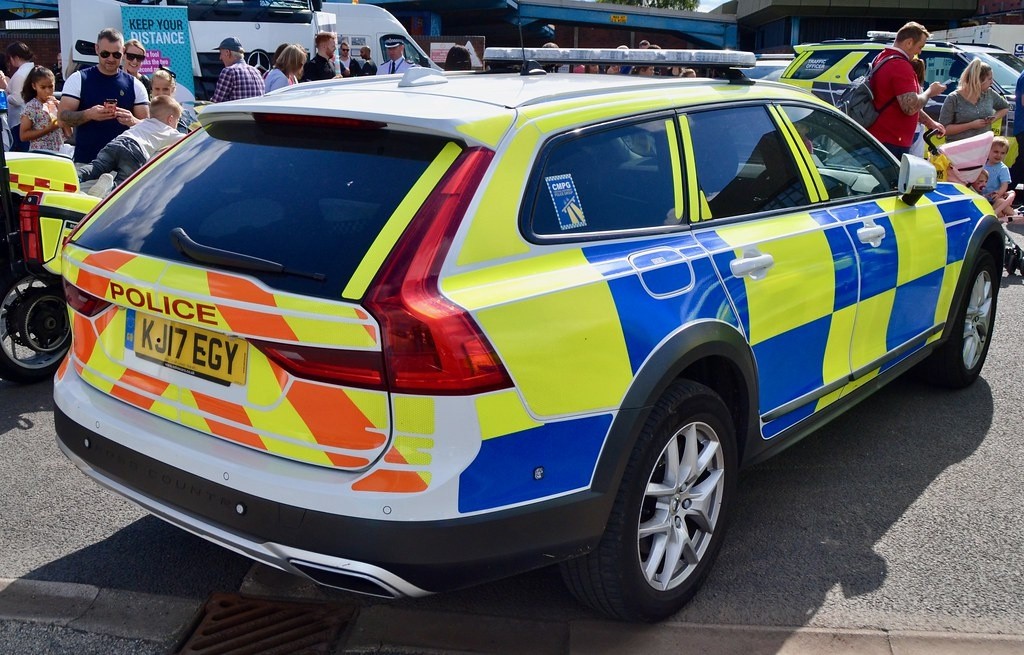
[740,32,1024,168]
[52,46,1006,626]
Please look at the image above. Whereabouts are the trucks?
[58,0,445,102]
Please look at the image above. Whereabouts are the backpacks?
[834,55,904,128]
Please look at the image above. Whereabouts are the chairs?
[949,61,966,78]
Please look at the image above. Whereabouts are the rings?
[126,118,128,121]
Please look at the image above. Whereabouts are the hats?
[213,37,242,51]
[382,34,406,47]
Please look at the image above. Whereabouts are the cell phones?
[942,80,952,86]
[986,116,996,121]
[105,99,117,117]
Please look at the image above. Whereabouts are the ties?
[391,62,395,74]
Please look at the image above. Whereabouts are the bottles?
[982,187,991,201]
[47,99,58,125]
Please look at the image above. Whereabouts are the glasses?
[342,48,349,52]
[126,53,145,62]
[98,47,121,59]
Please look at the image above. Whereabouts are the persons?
[863,22,1024,220]
[793,121,813,154]
[0,28,421,199]
[540,38,697,155]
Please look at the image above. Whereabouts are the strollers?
[923,128,1024,276]
